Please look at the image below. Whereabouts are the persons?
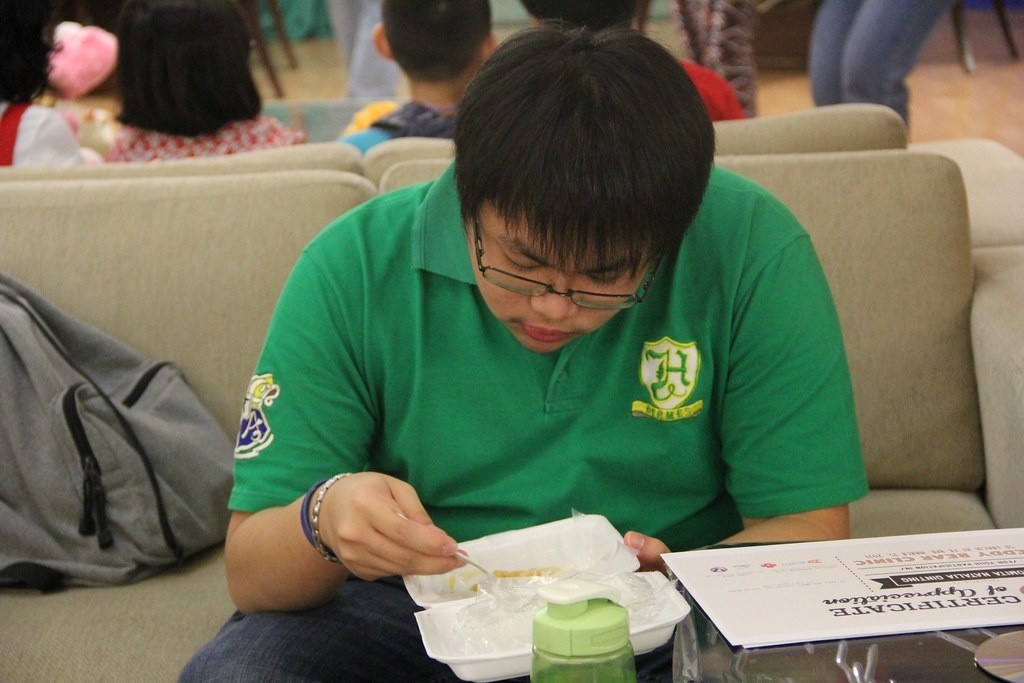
[176,18,871,683]
[806,1,950,130]
[0,0,757,169]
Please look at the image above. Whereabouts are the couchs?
[1,104,1024,246]
[1,149,1024,683]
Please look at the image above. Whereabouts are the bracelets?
[312,472,352,567]
[302,478,327,549]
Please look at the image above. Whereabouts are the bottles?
[532,578,641,683]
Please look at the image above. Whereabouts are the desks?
[671,540,1023,683]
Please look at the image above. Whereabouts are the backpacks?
[0,275,234,594]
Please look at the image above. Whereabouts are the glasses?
[472,211,657,311]
